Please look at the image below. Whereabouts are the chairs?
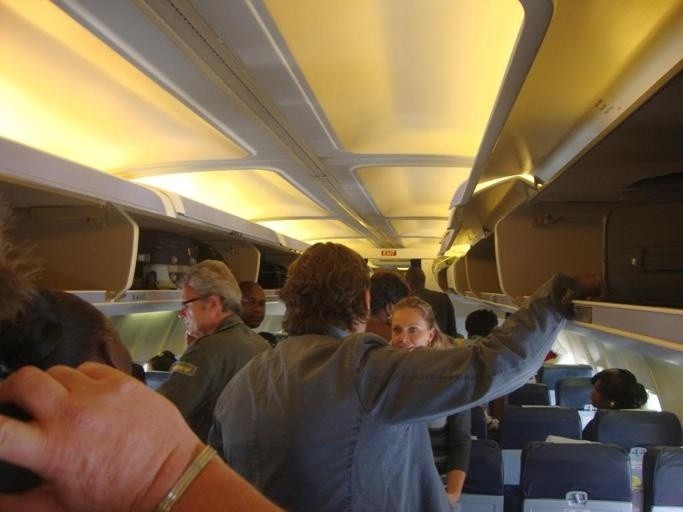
[143,371,172,387]
[431,364,683,511]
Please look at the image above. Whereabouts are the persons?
[1,241,607,511]
[581,368,647,440]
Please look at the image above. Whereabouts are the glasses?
[180,295,208,309]
